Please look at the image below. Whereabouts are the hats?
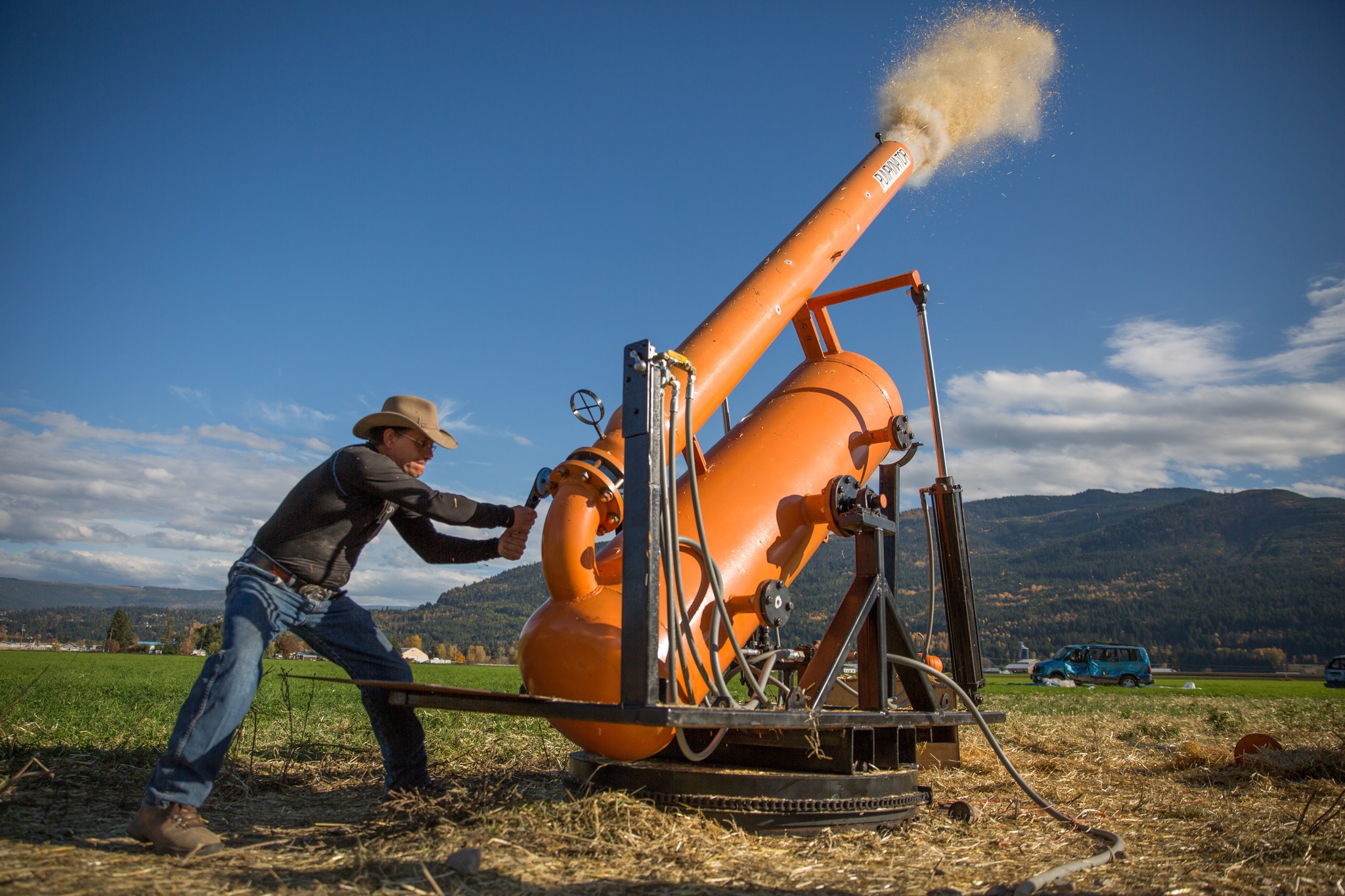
[352,395,457,449]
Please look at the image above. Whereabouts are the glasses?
[393,430,437,456]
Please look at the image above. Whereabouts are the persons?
[132,395,537,856]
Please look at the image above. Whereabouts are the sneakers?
[385,780,447,803]
[127,797,222,856]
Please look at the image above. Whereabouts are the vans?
[1323,655,1345,688]
[1031,643,1152,689]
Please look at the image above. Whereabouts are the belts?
[245,548,349,602]
